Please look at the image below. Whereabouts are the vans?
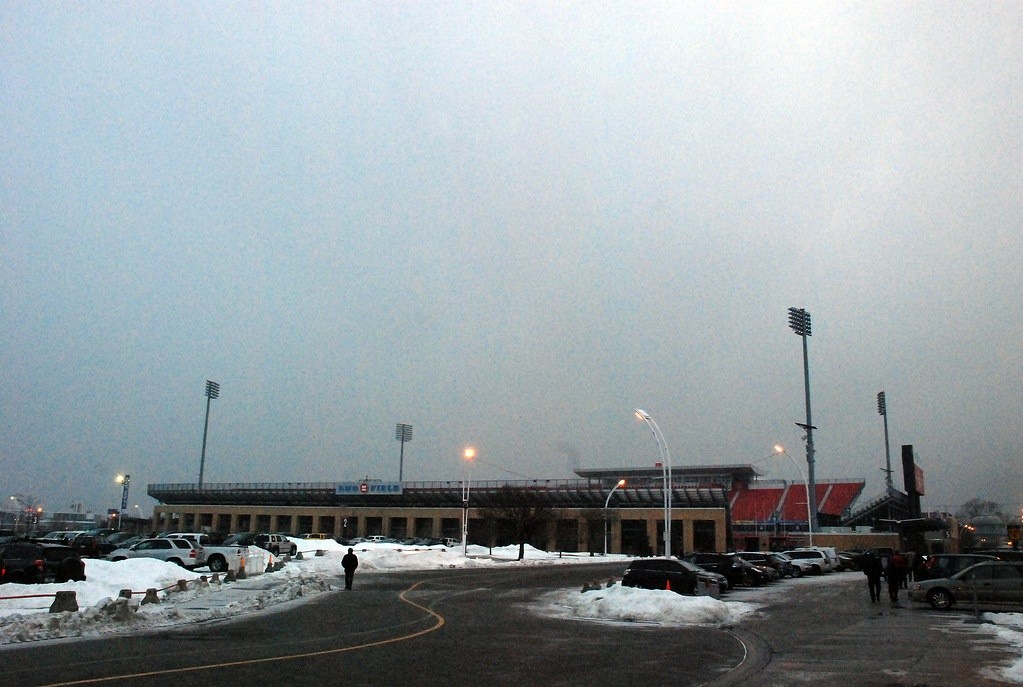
[735,545,895,580]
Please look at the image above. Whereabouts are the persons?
[863,553,883,603]
[862,548,937,587]
[883,558,902,603]
[341,548,358,591]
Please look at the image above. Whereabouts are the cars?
[623,559,729,598]
[299,532,460,549]
[29,529,297,559]
[908,561,1023,610]
[917,553,1000,580]
[678,552,772,587]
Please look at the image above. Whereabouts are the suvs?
[1,538,87,587]
[106,537,206,573]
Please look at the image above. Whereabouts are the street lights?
[461,447,475,556]
[115,475,124,531]
[9,495,21,539]
[787,307,818,533]
[877,391,896,534]
[602,480,626,557]
[774,446,812,547]
[197,380,220,503]
[395,422,413,489]
[633,409,671,560]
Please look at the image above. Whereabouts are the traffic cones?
[665,579,672,590]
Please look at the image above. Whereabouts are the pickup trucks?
[161,533,251,572]
